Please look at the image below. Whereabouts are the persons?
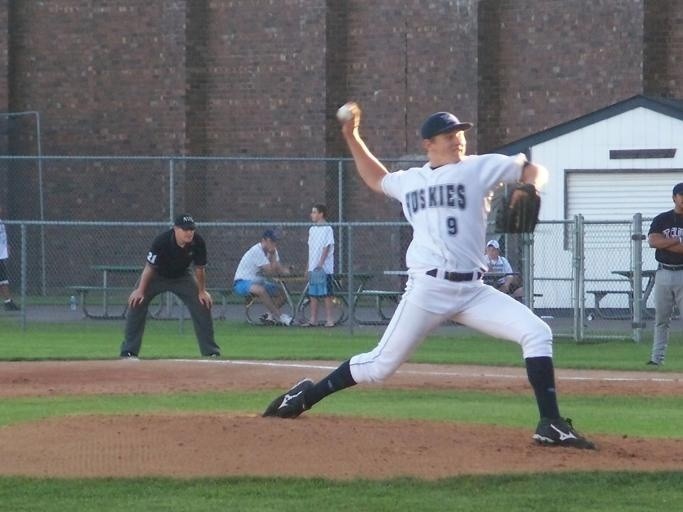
[268,102,597,449]
[0,204,17,311]
[482,240,513,293]
[301,203,337,327]
[647,184,682,367]
[230,230,288,326]
[117,213,221,359]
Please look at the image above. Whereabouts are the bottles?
[70,295,77,311]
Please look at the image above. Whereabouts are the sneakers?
[118,350,139,361]
[201,352,223,359]
[257,311,277,326]
[644,360,662,367]
[277,313,295,327]
[532,418,597,451]
[299,319,321,328]
[4,300,19,311]
[323,319,337,328]
[261,378,316,421]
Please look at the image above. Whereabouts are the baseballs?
[338,104,353,122]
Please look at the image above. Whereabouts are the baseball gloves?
[493,185,541,234]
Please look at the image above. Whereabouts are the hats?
[421,110,476,139]
[672,182,683,196]
[264,230,281,243]
[171,215,197,230]
[485,239,500,249]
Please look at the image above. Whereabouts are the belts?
[419,261,487,282]
